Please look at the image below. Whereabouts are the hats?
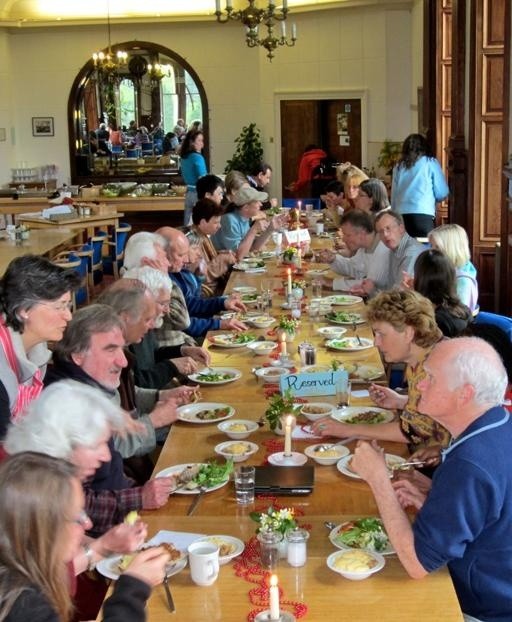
[233,188,269,207]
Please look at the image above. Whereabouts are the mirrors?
[67,41,210,188]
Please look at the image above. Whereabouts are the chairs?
[102,132,167,167]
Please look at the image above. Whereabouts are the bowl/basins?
[246,341,279,357]
[251,316,277,328]
[301,404,334,421]
[304,443,351,467]
[218,419,260,438]
[318,326,346,339]
[320,549,385,583]
[191,533,244,564]
[214,441,260,462]
[255,367,290,383]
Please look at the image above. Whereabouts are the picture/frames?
[31,116,54,137]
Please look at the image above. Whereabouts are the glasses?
[25,298,73,309]
[66,511,89,525]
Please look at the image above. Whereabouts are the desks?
[7,179,59,190]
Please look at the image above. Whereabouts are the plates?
[174,402,236,424]
[91,547,187,585]
[329,514,410,554]
[234,287,257,293]
[221,311,263,323]
[330,407,395,423]
[325,336,374,352]
[151,463,231,494]
[188,366,242,384]
[208,333,256,348]
[326,294,363,306]
[337,453,408,481]
[324,312,368,325]
[305,362,386,383]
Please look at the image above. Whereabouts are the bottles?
[258,525,314,569]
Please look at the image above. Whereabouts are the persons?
[78,117,200,157]
[0,129,277,622]
[286,132,512,622]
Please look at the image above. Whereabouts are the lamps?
[215,0,291,46]
[92,15,128,74]
[147,50,171,84]
[244,0,297,61]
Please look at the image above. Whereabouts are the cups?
[232,468,258,506]
[335,380,352,408]
[184,543,223,585]
